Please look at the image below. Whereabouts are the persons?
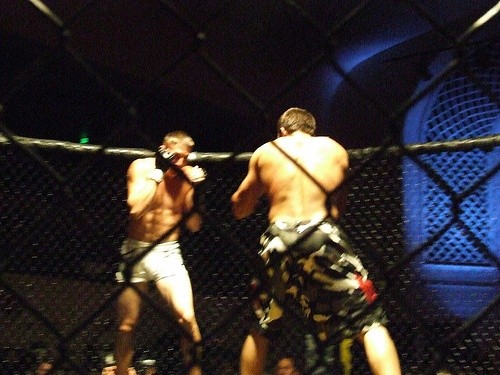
[276,358,299,375]
[230,106,401,375]
[26,349,57,375]
[116,131,205,375]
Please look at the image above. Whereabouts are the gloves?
[148,145,177,183]
[189,165,207,197]
